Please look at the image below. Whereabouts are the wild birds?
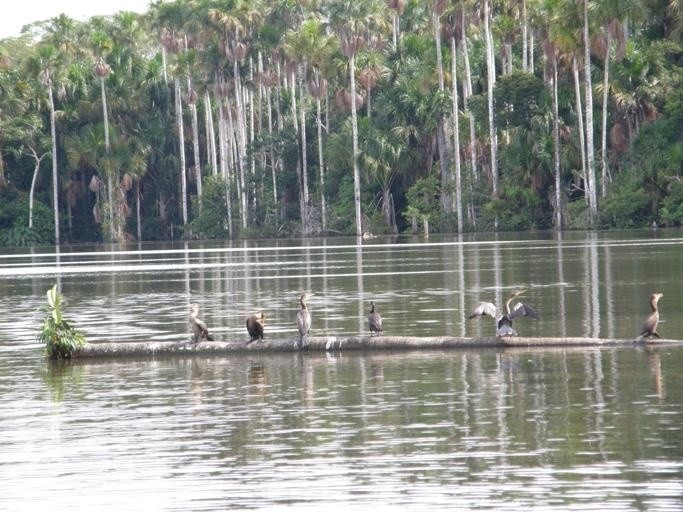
[185,302,216,345]
[245,311,267,342]
[634,292,664,342]
[368,301,385,337]
[468,288,541,338]
[294,293,313,348]
